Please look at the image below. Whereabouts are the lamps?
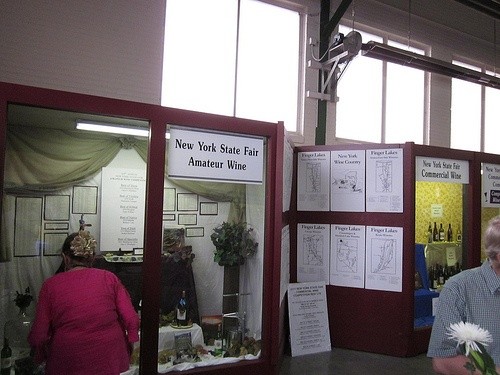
[352,0,500,90]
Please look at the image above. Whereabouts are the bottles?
[0,337,12,375]
[448,224,452,242]
[176,290,189,326]
[429,262,462,289]
[428,221,432,243]
[439,223,444,241]
[456,230,462,242]
[213,325,223,356]
[433,222,438,241]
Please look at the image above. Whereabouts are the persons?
[28,230,139,375]
[427,215,500,375]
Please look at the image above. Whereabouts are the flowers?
[446,320,499,375]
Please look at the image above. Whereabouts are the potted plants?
[210,219,258,266]
